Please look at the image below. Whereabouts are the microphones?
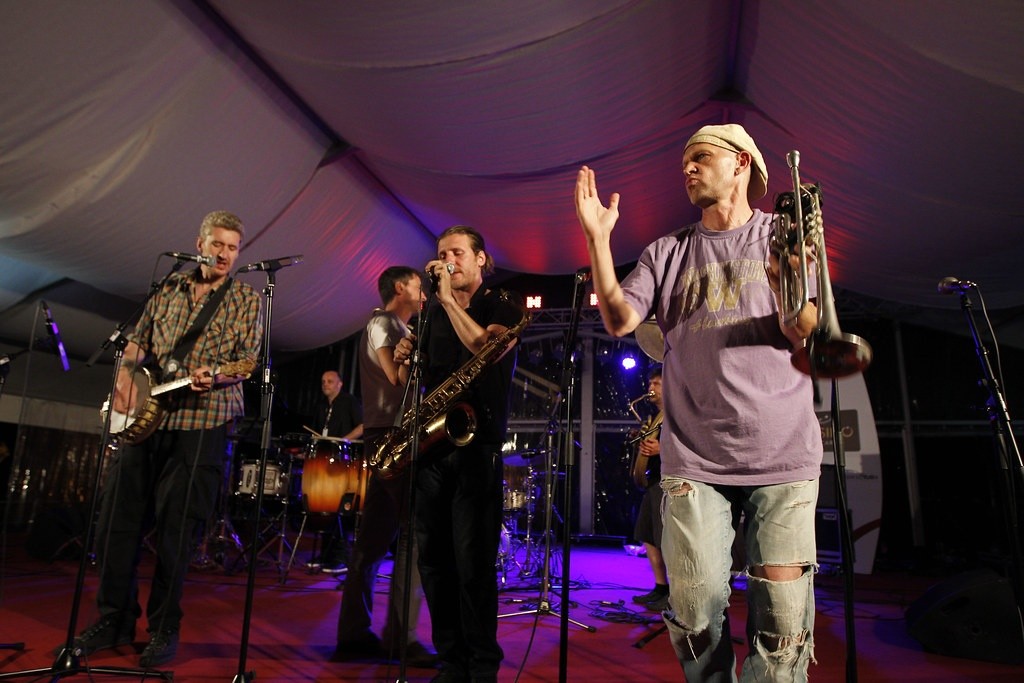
[574,266,592,285]
[425,263,455,279]
[164,252,215,266]
[938,276,979,297]
[239,255,304,272]
[40,300,71,372]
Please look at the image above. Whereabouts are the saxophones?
[367,287,533,480]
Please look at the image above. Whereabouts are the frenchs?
[630,315,664,490]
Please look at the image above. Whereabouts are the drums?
[340,439,372,517]
[279,433,311,454]
[502,486,536,512]
[238,458,287,500]
[301,435,351,518]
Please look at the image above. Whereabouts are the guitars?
[100,356,256,448]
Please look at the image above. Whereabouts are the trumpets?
[770,150,872,378]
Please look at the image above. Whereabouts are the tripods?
[636,619,744,649]
[497,402,596,633]
[0,259,190,683]
[186,447,306,575]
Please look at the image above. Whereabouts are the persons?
[55,210,265,667]
[574,123,835,682]
[631,366,670,610]
[331,223,526,683]
[306,371,364,573]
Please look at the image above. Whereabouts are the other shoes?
[373,641,439,667]
[632,590,671,610]
[307,557,348,573]
[334,633,382,660]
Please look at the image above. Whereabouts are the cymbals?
[502,453,545,466]
[226,433,279,441]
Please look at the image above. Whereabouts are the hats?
[683,124,768,202]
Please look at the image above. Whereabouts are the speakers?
[814,508,856,565]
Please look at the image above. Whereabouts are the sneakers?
[72,619,135,653]
[140,629,179,667]
